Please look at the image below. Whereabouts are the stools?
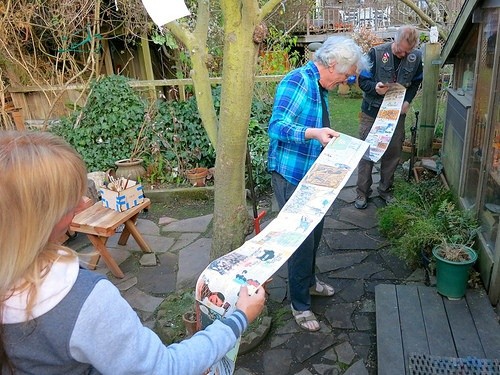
[70,198,151,278]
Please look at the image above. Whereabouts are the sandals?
[310,280,335,296]
[290,303,321,332]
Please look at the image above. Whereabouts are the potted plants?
[115,100,162,181]
[431,125,444,155]
[376,167,489,298]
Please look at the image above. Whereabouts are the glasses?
[395,44,412,54]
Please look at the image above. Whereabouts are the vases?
[182,312,197,335]
[187,167,208,187]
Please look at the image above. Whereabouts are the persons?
[0,129,266,375]
[354,24,423,209]
[268,36,374,331]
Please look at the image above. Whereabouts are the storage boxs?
[99,184,144,212]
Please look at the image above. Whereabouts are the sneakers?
[355,195,368,209]
[376,187,394,200]
